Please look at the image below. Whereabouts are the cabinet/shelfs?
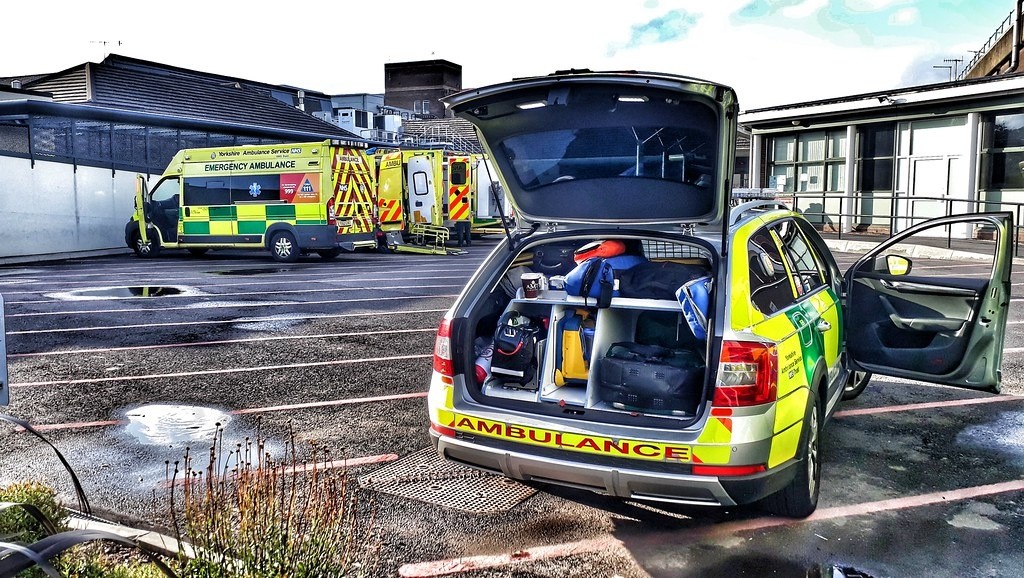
[482,294,696,421]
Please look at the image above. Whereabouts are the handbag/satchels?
[574,240,625,265]
[675,274,715,340]
[620,261,708,301]
[499,266,534,299]
[600,341,706,417]
[563,254,648,308]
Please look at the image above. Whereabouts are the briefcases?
[533,240,592,276]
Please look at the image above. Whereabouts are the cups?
[520,274,540,300]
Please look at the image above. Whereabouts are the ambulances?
[125,140,380,262]
[366,149,474,256]
[453,153,521,240]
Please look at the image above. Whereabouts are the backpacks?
[490,323,539,387]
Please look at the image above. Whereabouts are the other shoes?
[467,242,471,247]
[458,244,462,247]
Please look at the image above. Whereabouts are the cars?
[426,67,1015,519]
